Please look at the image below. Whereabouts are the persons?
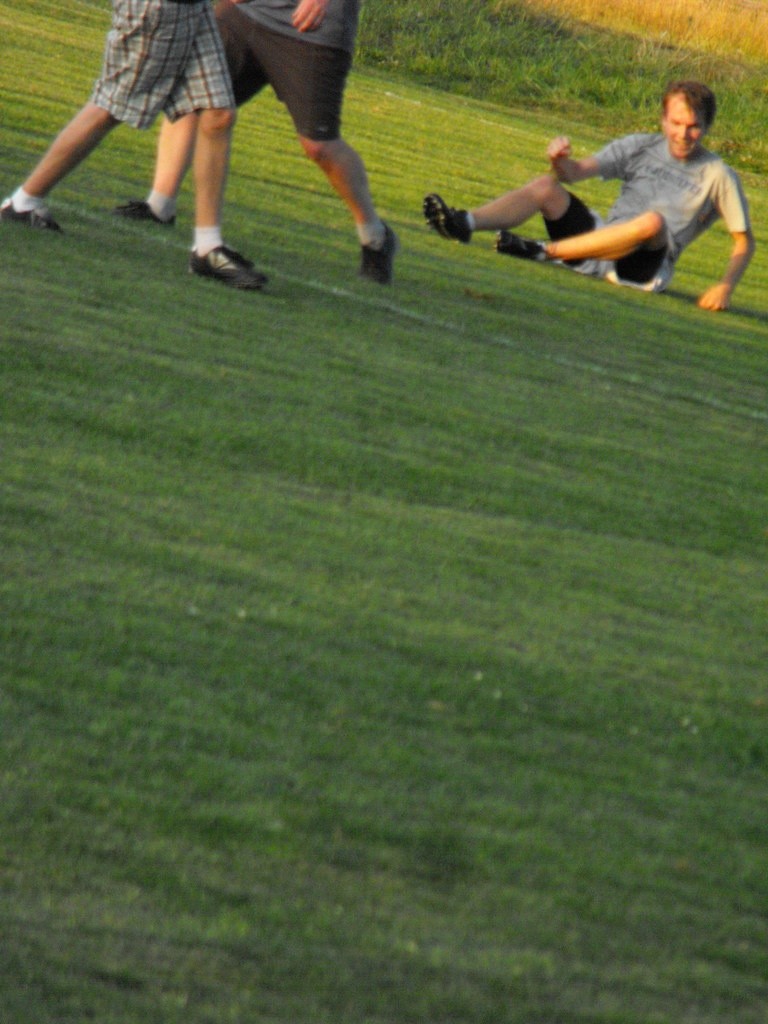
[423,81,756,311]
[0,0,399,290]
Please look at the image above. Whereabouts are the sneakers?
[0,198,62,231]
[493,228,546,263]
[189,244,269,293]
[360,221,398,286]
[424,192,472,245]
[113,202,177,229]
